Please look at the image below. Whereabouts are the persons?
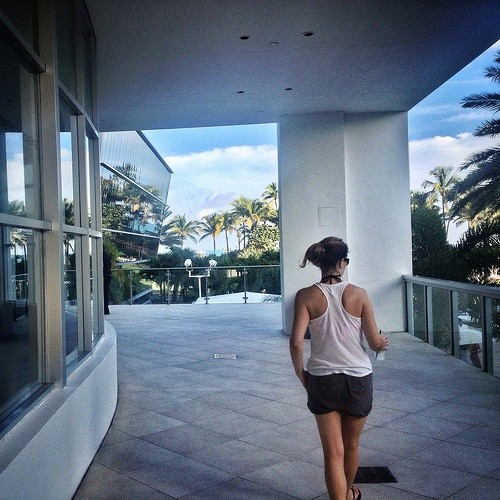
[458,318,483,368]
[290,236,388,500]
[102,244,111,315]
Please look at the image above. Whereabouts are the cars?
[458,311,478,326]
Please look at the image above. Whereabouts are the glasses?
[343,258,350,265]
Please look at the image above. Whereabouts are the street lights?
[183,257,217,300]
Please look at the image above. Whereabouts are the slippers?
[350,485,361,500]
